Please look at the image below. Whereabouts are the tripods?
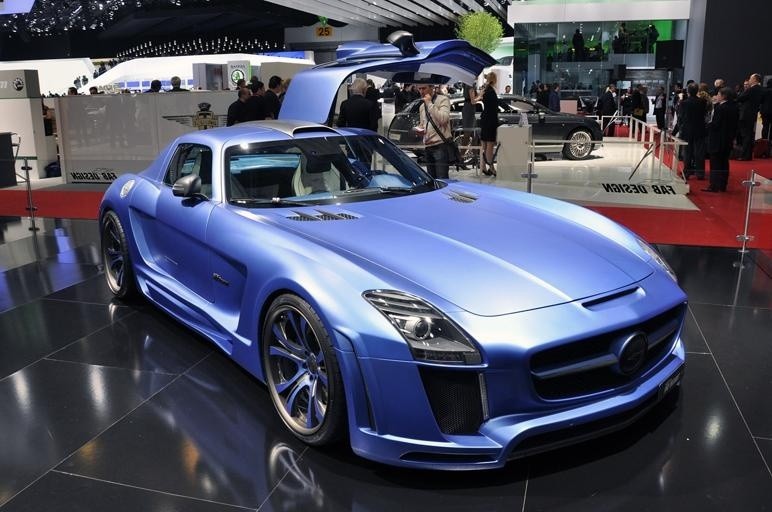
[627,72,687,186]
[603,83,629,135]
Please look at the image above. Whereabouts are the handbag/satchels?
[447,140,462,164]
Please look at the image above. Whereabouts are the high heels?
[455,158,480,170]
[483,168,496,177]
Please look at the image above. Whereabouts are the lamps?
[116,36,286,57]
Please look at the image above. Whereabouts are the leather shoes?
[701,187,715,192]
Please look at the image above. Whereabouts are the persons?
[226,75,292,127]
[336,71,498,180]
[573,25,659,61]
[41,55,190,98]
[597,73,772,192]
[505,85,511,93]
[529,80,560,112]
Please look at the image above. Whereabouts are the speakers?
[613,63,626,79]
[654,39,684,69]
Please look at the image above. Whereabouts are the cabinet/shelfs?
[496,124,532,180]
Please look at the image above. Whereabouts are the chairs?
[192,150,247,200]
[292,154,350,195]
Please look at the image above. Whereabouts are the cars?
[99,30,688,471]
[388,94,603,164]
